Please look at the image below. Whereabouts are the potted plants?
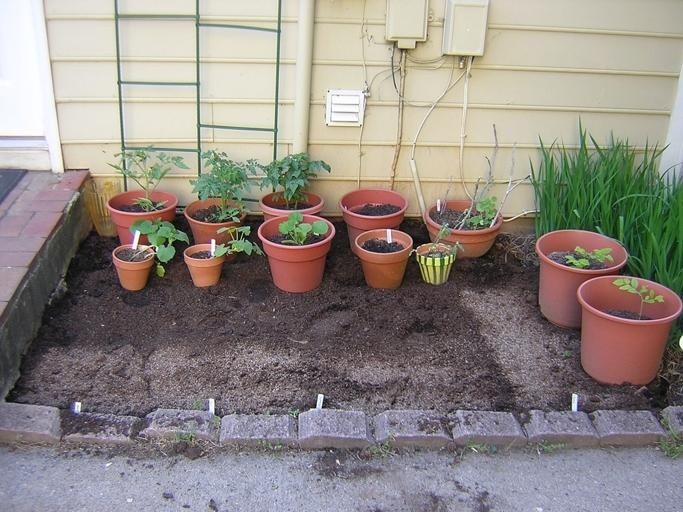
[108,216,190,291]
[256,210,337,291]
[423,145,504,259]
[183,225,266,289]
[338,187,409,249]
[535,228,683,390]
[107,144,191,243]
[250,151,333,219]
[413,222,463,286]
[353,227,414,289]
[183,145,259,245]
